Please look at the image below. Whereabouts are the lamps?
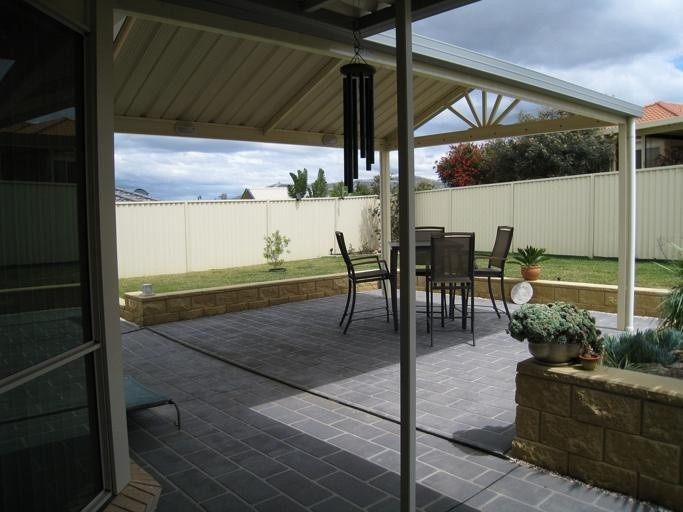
[340,27,376,193]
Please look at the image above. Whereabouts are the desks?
[391,241,467,331]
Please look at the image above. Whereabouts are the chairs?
[456,225,515,320]
[414,226,442,316]
[425,232,476,347]
[124,376,180,431]
[334,231,397,335]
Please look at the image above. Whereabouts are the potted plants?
[505,245,551,281]
[578,334,605,371]
[505,302,596,366]
